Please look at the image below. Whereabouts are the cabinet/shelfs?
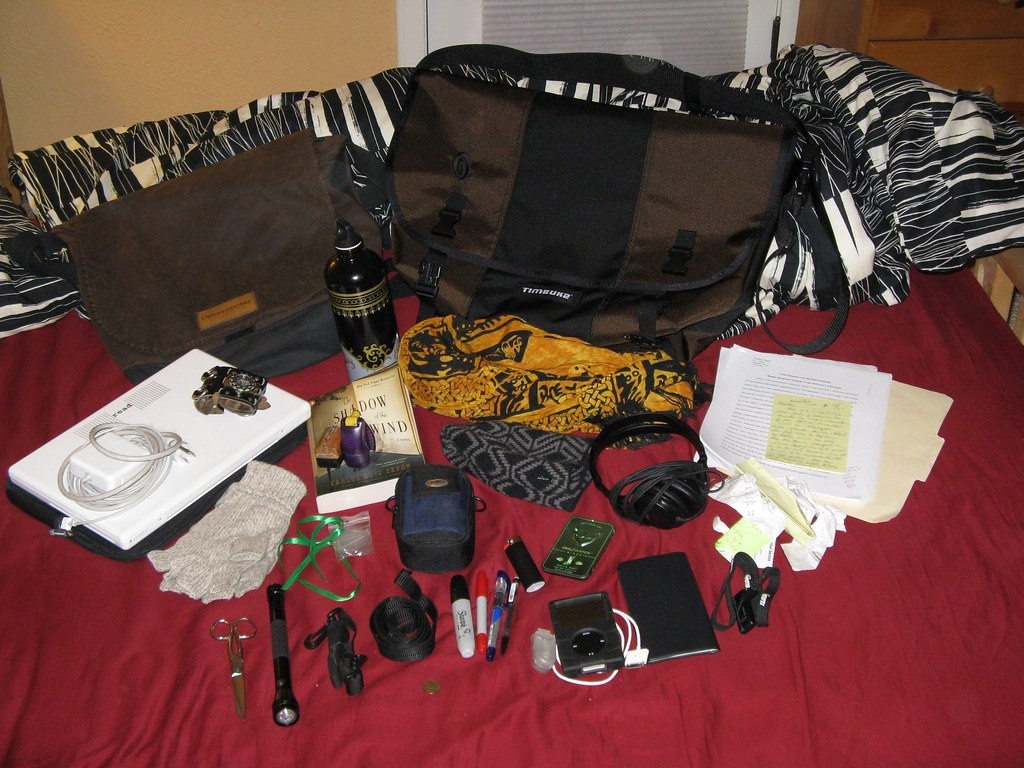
[795,0,1024,103]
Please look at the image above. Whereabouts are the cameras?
[548,591,625,680]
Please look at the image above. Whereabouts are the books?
[617,552,720,663]
[306,361,425,515]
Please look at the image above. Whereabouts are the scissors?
[210,615,257,719]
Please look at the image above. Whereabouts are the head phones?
[588,413,724,530]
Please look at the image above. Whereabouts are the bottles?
[326,219,402,385]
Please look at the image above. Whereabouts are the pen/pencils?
[475,569,520,662]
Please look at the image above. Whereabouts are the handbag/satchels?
[386,43,848,361]
[11,130,385,386]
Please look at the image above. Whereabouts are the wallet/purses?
[618,552,720,666]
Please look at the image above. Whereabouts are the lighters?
[504,534,545,593]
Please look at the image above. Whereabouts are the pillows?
[0,183,82,341]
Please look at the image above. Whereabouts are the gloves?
[148,461,307,604]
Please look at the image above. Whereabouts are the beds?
[1,264,1024,767]
